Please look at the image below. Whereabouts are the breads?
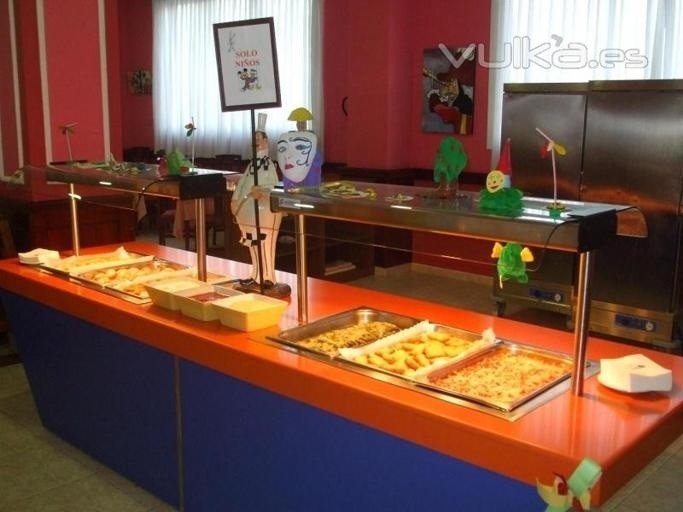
[353,330,473,375]
[87,261,176,283]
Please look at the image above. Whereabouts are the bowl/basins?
[143,276,289,333]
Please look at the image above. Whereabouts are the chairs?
[124,146,250,250]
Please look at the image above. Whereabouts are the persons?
[231,131,283,287]
